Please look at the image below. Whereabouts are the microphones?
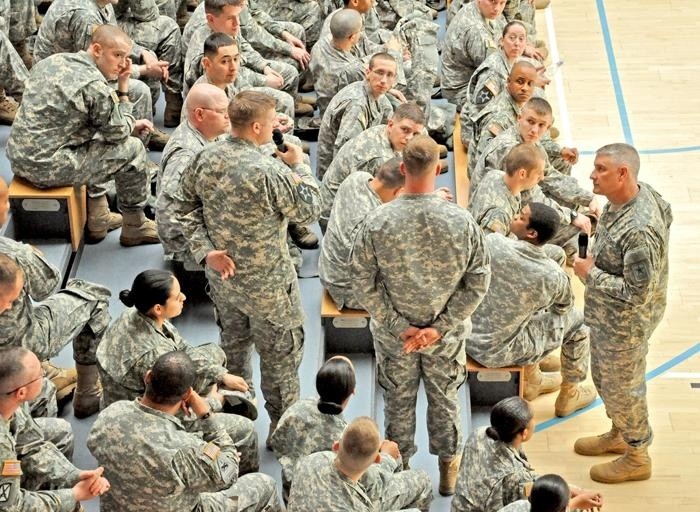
[272,129,288,154]
[577,232,588,259]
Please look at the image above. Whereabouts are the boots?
[590,444,652,484]
[41,360,78,400]
[87,195,123,240]
[120,210,161,247]
[438,456,462,496]
[522,354,562,401]
[73,364,103,419]
[0,87,20,124]
[555,380,596,417]
[13,39,33,67]
[575,422,628,456]
[164,92,183,128]
[290,66,318,118]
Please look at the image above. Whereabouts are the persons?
[272,355,433,511]
[2,0,187,246]
[1,178,110,511]
[349,133,490,496]
[309,0,456,311]
[155,0,326,271]
[453,397,602,512]
[176,91,322,447]
[440,0,600,417]
[571,141,673,483]
[88,268,281,512]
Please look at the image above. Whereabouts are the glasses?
[6,369,46,395]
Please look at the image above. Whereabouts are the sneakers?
[289,223,319,249]
[437,144,449,175]
[149,126,170,152]
